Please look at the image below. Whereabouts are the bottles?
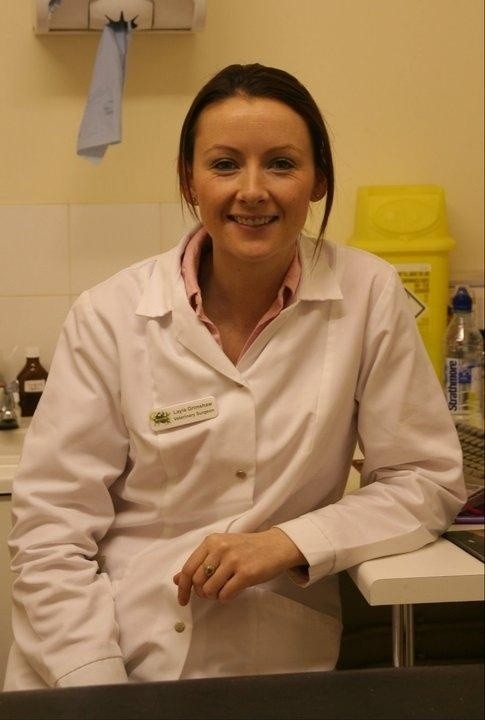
[441,286,483,422]
[17,349,52,416]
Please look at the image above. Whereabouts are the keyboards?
[454,419,485,512]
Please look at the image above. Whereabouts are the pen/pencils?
[454,515,485,524]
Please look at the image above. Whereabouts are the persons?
[2,61,471,692]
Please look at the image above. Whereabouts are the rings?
[201,562,216,577]
